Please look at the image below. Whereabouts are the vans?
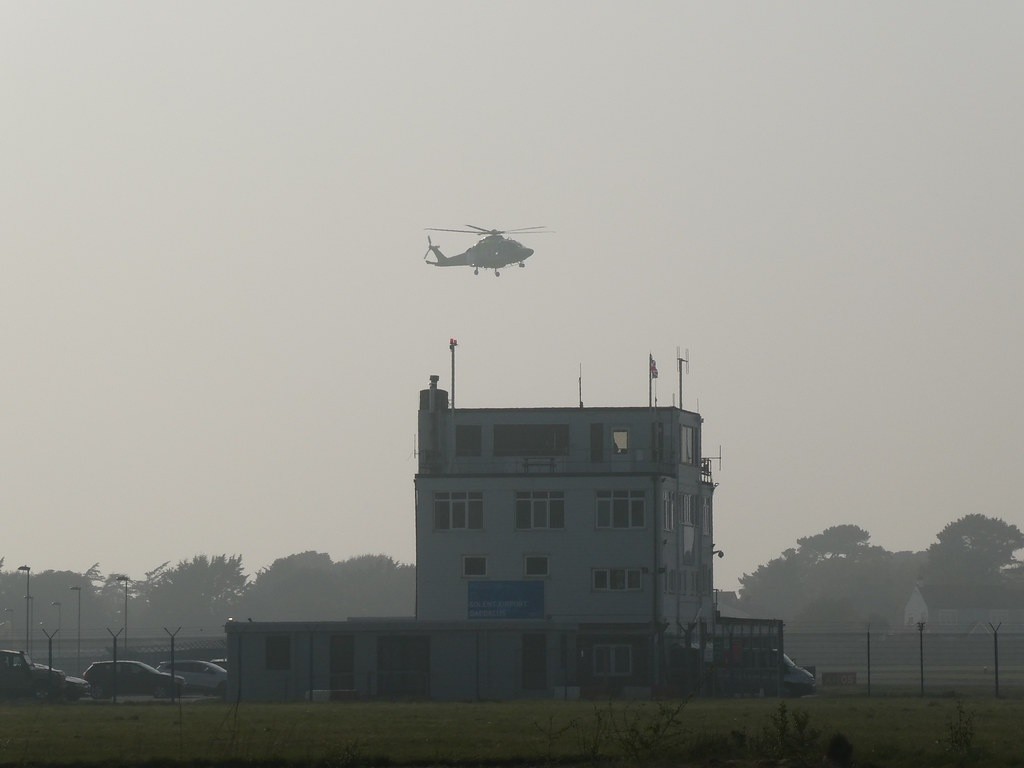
[722,648,816,699]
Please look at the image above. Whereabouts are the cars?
[16,663,90,701]
[83,660,187,700]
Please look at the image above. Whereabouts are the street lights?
[18,566,31,652]
[116,576,128,660]
[52,602,61,661]
[70,587,81,670]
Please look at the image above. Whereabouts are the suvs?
[0,650,66,700]
[157,659,229,693]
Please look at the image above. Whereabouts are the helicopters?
[424,224,554,277]
